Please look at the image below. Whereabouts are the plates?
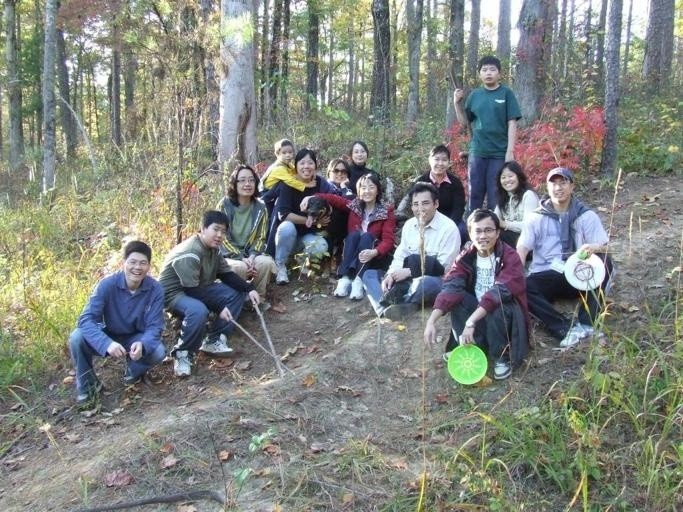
[447,344,487,386]
[564,251,605,291]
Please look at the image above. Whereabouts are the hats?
[546,168,574,182]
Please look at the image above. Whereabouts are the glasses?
[475,227,497,235]
[126,259,148,268]
[236,178,256,184]
[330,168,348,175]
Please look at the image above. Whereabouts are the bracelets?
[504,219,509,232]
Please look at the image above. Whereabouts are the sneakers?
[349,279,365,301]
[76,381,104,405]
[333,278,351,297]
[123,353,142,387]
[198,333,234,358]
[560,323,604,346]
[382,303,418,320]
[174,350,192,377]
[494,361,512,379]
[275,266,289,284]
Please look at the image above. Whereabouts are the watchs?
[463,320,478,329]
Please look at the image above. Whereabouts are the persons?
[453,55,522,213]
[515,169,614,348]
[392,144,468,253]
[254,138,377,285]
[423,207,531,381]
[300,174,395,300]
[69,241,167,404]
[491,161,540,260]
[362,180,461,322]
[214,165,273,312]
[159,210,260,376]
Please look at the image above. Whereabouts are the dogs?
[304,197,350,279]
